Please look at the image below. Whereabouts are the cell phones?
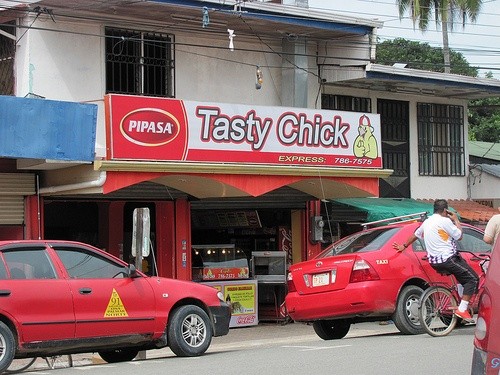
[446,210,453,216]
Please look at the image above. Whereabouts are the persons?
[391,198,480,325]
[483,204,500,251]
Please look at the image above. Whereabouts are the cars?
[471,229,499,375]
[284,211,491,341]
[0,238,232,373]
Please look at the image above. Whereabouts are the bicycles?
[6,354,73,374]
[418,251,494,337]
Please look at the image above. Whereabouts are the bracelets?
[404,244,408,248]
